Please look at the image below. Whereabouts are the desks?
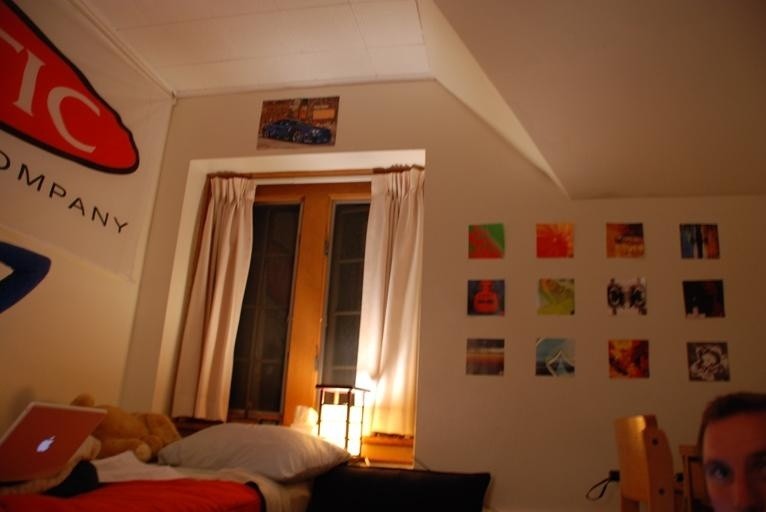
[679,445,707,512]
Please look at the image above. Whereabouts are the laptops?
[1,401,109,483]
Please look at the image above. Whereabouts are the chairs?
[613,415,682,512]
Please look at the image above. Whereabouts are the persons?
[696,390,765,512]
[472,281,499,314]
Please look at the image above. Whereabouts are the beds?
[0,463,311,512]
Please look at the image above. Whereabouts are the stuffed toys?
[71,394,181,461]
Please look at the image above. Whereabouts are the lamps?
[316,385,370,463]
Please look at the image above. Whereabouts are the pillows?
[156,422,350,484]
[306,467,491,512]
